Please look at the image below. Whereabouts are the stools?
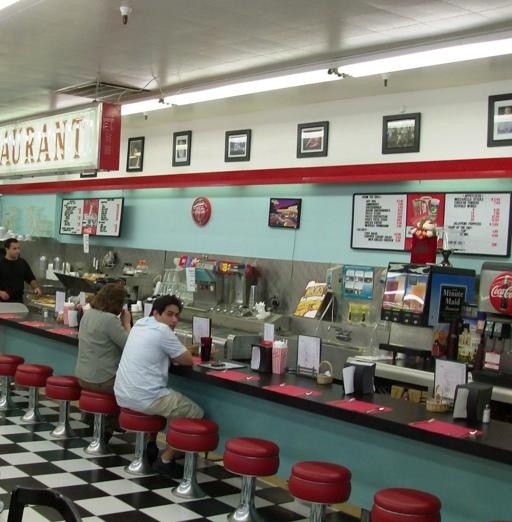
[286,461,353,521]
[221,436,280,522]
[0,354,166,479]
[366,487,442,521]
[166,415,220,500]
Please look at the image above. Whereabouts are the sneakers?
[153,456,184,479]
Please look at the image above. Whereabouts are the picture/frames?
[79,172,98,177]
[295,120,330,160]
[222,128,251,163]
[126,136,146,172]
[171,129,192,167]
[486,92,512,147]
[380,110,422,155]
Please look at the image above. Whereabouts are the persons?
[0,238,42,305]
[113,295,205,475]
[75,282,132,445]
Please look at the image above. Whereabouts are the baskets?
[317,361,333,384]
[427,385,448,412]
[183,334,199,353]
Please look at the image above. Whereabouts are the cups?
[64,303,74,324]
[156,259,181,302]
[412,196,438,221]
[271,348,288,374]
[131,300,142,312]
[201,336,212,361]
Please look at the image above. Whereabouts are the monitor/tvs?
[53,272,95,294]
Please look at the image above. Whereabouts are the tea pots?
[120,263,134,275]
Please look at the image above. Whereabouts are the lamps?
[121,37,512,117]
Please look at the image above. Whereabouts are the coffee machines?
[215,260,256,308]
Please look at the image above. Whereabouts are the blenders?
[192,260,223,307]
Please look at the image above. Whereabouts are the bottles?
[42,307,49,319]
[248,285,256,307]
[481,403,490,423]
[445,333,459,360]
[136,259,148,274]
